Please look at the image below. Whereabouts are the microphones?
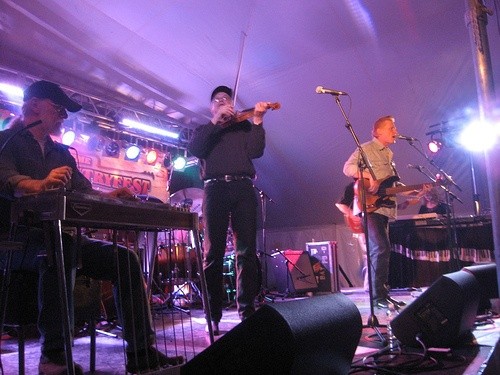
[316,86,348,95]
[398,135,419,141]
[406,163,426,169]
[0,119,42,155]
[298,274,310,280]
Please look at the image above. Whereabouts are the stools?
[0,268,101,375]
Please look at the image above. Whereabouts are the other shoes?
[125,346,184,374]
[39,357,83,375]
[205,318,218,335]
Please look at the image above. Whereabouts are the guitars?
[346,213,363,234]
[353,173,452,213]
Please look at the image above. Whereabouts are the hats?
[211,86,233,99]
[24,81,82,113]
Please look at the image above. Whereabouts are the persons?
[186,86,267,335]
[1,79,188,374]
[336,116,405,308]
[418,184,452,217]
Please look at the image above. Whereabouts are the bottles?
[385,303,399,341]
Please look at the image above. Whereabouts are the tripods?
[334,95,390,346]
[154,231,191,315]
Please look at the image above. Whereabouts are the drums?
[221,255,235,276]
[157,242,200,284]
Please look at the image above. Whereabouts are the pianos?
[13,190,218,375]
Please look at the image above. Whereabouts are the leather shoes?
[375,299,400,309]
[387,296,406,306]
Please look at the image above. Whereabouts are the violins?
[218,101,281,128]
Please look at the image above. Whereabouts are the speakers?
[270,250,318,295]
[179,291,364,375]
[390,263,498,348]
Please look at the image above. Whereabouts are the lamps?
[426,131,443,153]
[86,133,120,158]
[60,127,76,145]
[144,147,188,171]
[121,142,140,161]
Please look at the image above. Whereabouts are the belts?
[204,174,251,184]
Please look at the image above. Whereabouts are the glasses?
[211,96,231,102]
[38,98,66,115]
